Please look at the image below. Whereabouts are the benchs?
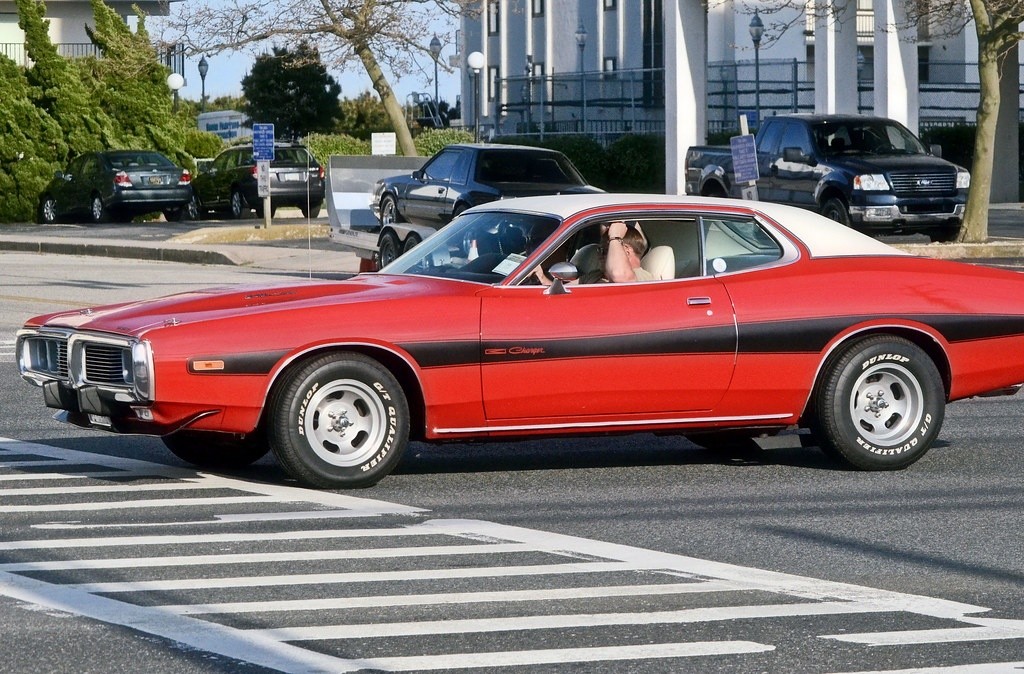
[685,256,781,277]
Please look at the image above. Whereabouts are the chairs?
[570,244,676,280]
[817,137,877,150]
[111,162,159,167]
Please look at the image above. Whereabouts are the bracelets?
[607,236,623,244]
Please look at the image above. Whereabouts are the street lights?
[573,19,590,134]
[747,6,767,125]
[198,53,211,115]
[429,31,444,128]
[167,72,185,115]
[466,50,486,145]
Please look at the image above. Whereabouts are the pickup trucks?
[683,109,971,242]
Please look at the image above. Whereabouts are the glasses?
[596,247,608,257]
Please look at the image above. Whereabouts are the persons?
[524,219,653,285]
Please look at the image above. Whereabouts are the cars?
[38,141,326,225]
[13,192,1024,490]
[368,143,612,235]
